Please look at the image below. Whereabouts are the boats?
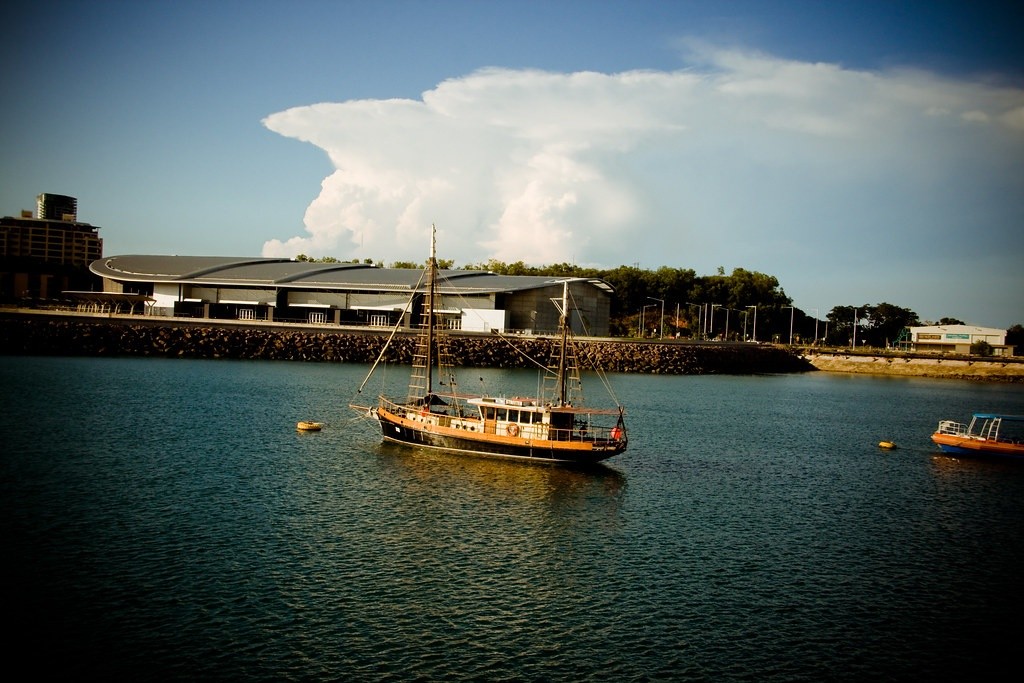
[349,222,629,471]
[930,414,1024,465]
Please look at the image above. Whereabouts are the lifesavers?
[505,423,520,437]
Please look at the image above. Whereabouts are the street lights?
[646,296,664,341]
[781,306,794,345]
[686,302,701,341]
[745,305,757,340]
[710,304,723,333]
[641,304,656,337]
[718,307,729,340]
[732,308,747,343]
